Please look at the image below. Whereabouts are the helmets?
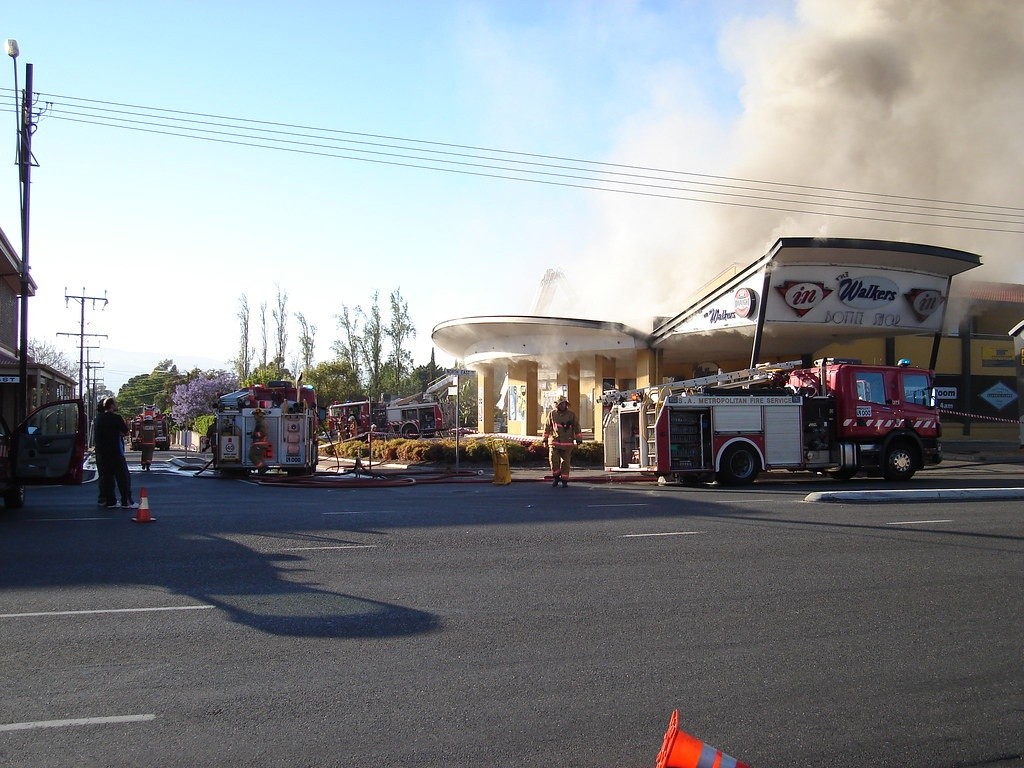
[554,394,571,407]
[252,409,265,415]
[350,416,355,420]
[143,415,153,421]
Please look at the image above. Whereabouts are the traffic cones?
[653,708,754,768]
[133,487,156,523]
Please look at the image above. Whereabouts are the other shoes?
[142,466,145,469]
[130,502,139,508]
[121,505,129,508]
[107,502,121,508]
[98,501,107,506]
[258,466,267,474]
[147,466,150,470]
[562,480,568,487]
[552,476,561,487]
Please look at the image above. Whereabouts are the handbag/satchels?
[133,436,143,451]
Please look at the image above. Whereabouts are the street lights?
[5,37,34,421]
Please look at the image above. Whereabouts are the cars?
[0,398,85,509]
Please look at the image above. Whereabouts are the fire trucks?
[200,379,319,478]
[129,402,171,450]
[329,373,452,438]
[596,357,944,487]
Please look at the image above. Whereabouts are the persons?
[249,409,270,474]
[368,424,380,442]
[348,416,357,441]
[206,418,217,468]
[139,415,159,471]
[94,397,139,509]
[542,395,582,487]
